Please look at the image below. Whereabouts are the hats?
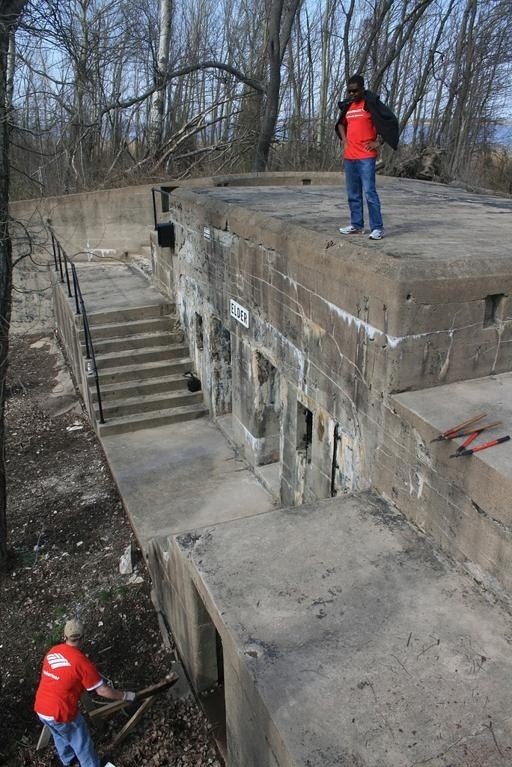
[65,619,85,640]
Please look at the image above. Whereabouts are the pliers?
[449,429,511,458]
[430,412,503,443]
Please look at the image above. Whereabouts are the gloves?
[123,690,136,701]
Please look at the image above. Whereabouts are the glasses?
[347,89,360,94]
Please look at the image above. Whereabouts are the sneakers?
[369,229,385,240]
[339,226,366,235]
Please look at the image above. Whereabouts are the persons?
[332,74,400,240]
[32,618,136,767]
